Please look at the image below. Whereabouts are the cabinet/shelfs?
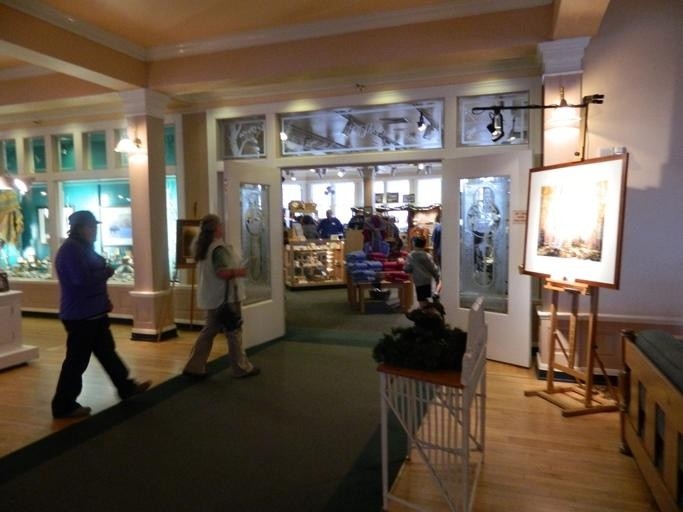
[283,241,344,288]
[344,262,416,313]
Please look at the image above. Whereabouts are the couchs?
[617,324,683,511]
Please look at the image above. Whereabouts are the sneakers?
[183,367,211,377]
[53,405,91,418]
[234,367,262,379]
[121,380,149,401]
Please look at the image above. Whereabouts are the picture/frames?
[521,151,630,290]
[36,204,76,244]
[173,219,198,268]
[100,203,133,248]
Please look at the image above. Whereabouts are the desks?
[376,297,488,512]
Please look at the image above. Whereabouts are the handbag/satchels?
[216,303,244,332]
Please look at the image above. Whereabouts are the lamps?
[280,85,606,203]
[0,173,13,190]
[112,137,141,154]
[13,176,35,193]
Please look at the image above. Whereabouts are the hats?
[68,211,100,227]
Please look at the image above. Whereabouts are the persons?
[403,236,441,307]
[183,215,261,383]
[296,209,344,239]
[51,211,151,419]
[431,216,443,299]
